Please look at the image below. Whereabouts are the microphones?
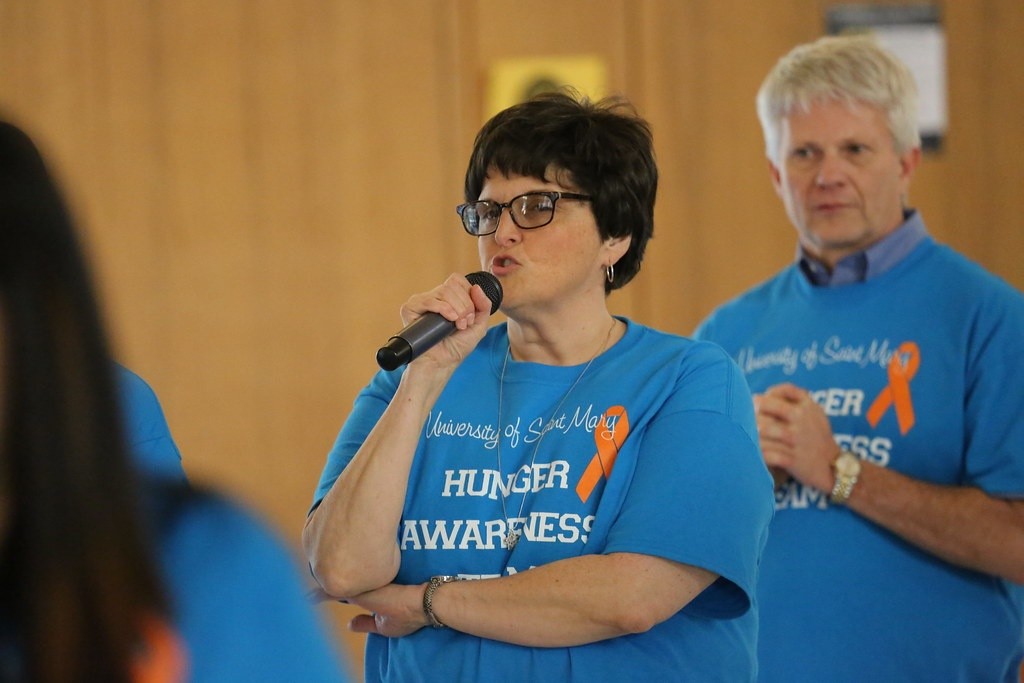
[376,271,503,370]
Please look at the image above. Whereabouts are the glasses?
[456,191,591,236]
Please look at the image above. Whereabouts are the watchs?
[423,575,457,630]
[829,450,863,505]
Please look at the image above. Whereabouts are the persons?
[1,116,358,683]
[695,33,1024,683]
[302,90,774,683]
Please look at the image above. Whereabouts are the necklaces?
[496,316,617,550]
